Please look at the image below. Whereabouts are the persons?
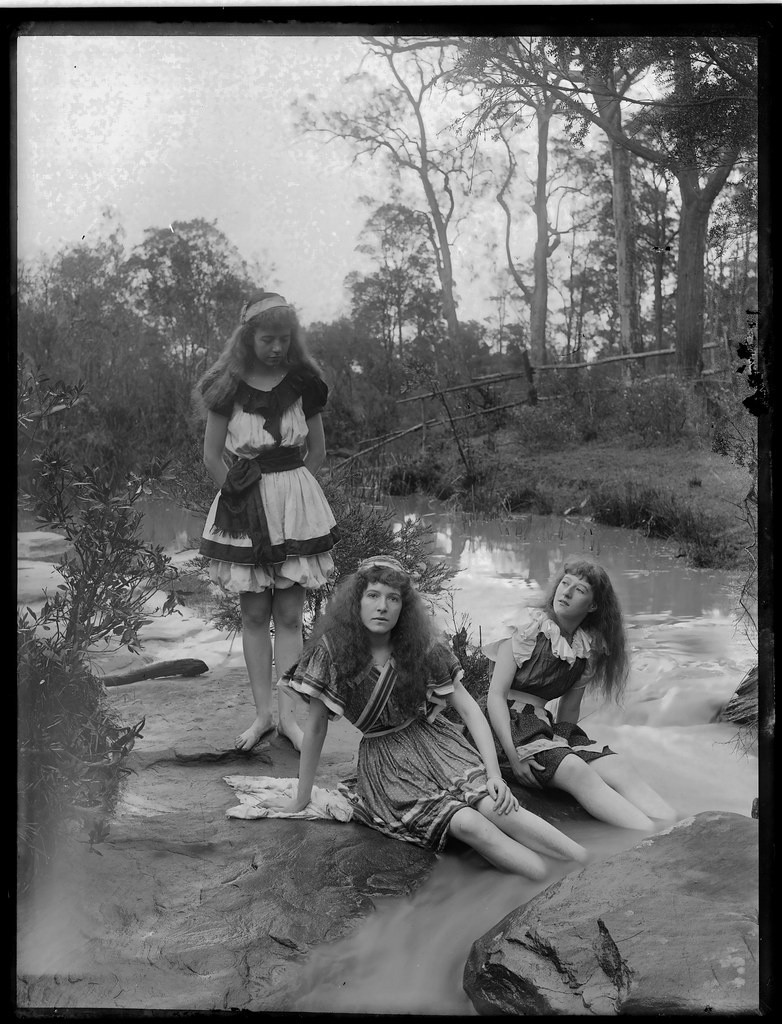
[255,555,589,884]
[188,288,342,753]
[462,560,677,830]
